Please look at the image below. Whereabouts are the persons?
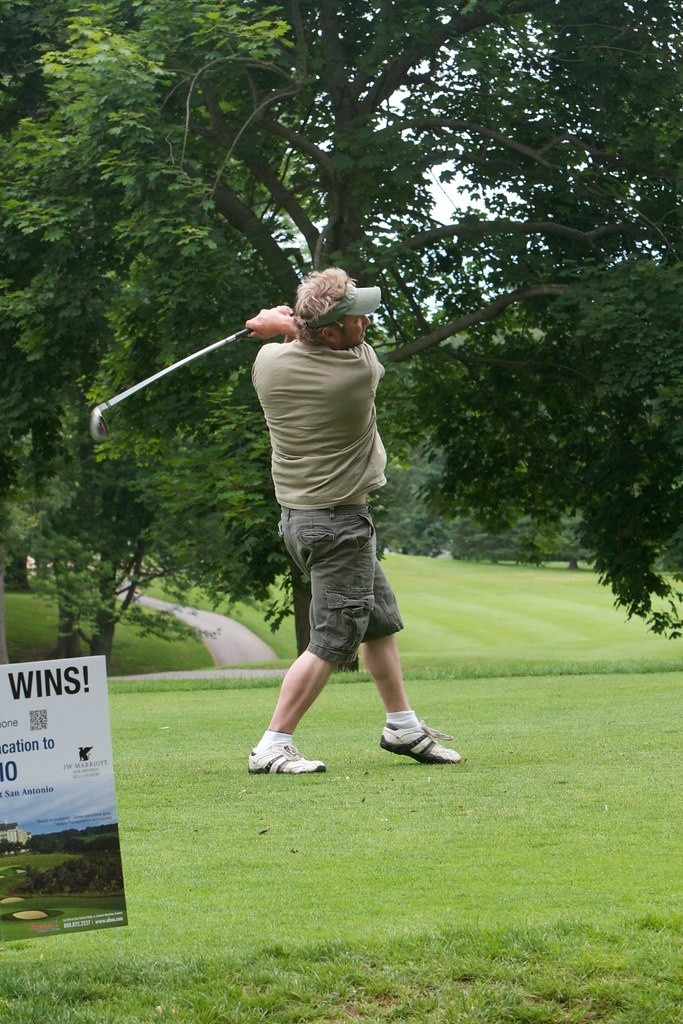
[246,267,462,773]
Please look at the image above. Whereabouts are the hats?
[305,277,381,328]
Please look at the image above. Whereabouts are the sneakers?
[247,742,327,775]
[379,720,462,765]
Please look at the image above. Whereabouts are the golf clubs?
[89,307,296,443]
